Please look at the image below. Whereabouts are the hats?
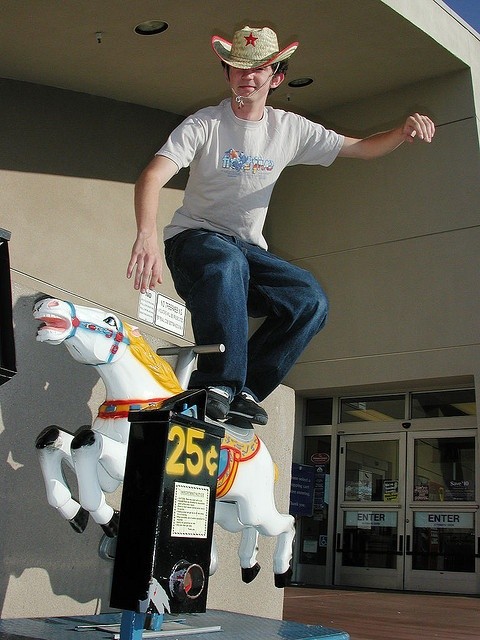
[212,25,299,71]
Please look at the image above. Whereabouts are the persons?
[126,26,436,425]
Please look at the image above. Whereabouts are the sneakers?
[230,391,268,425]
[205,385,231,419]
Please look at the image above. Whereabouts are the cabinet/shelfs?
[291,389,480,599]
